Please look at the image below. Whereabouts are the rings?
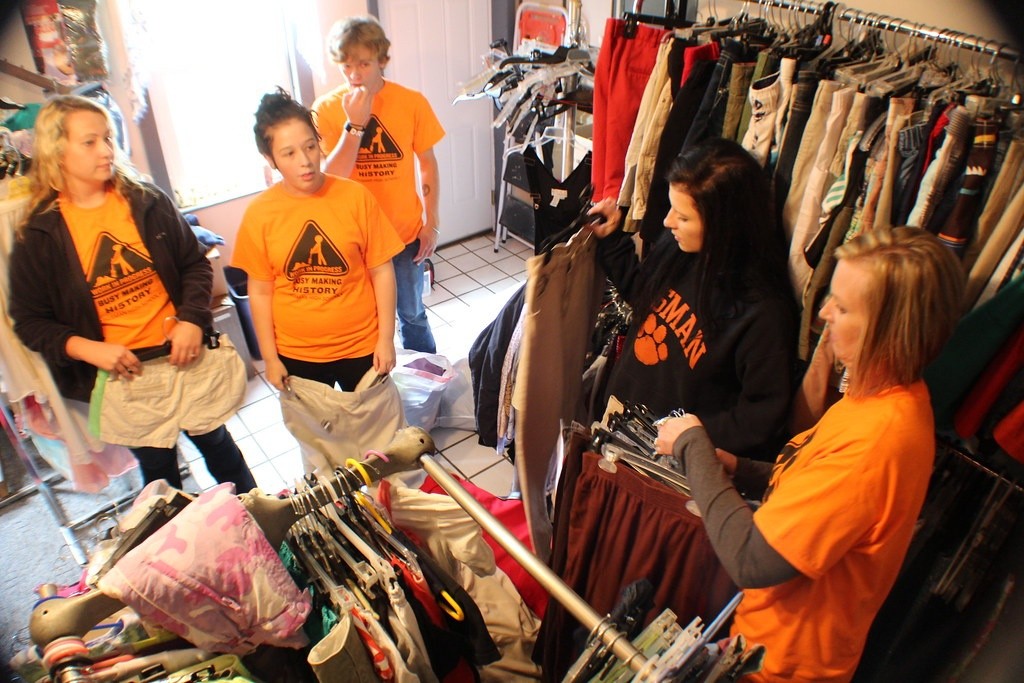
[188,352,196,358]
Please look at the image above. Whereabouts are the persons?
[587,138,800,463]
[231,85,405,478]
[311,15,445,354]
[8,96,257,495]
[655,226,967,683]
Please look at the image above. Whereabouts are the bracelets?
[426,223,440,234]
[344,121,365,137]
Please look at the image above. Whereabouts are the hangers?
[269,447,425,621]
[560,576,766,683]
[585,400,763,507]
[128,316,220,361]
[536,183,608,263]
[585,273,635,358]
[3,592,242,683]
[621,0,1023,126]
[0,124,37,182]
[904,441,1024,597]
[449,17,602,160]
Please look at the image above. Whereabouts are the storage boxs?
[203,246,228,310]
[211,294,255,381]
[222,264,261,361]
[421,262,431,298]
